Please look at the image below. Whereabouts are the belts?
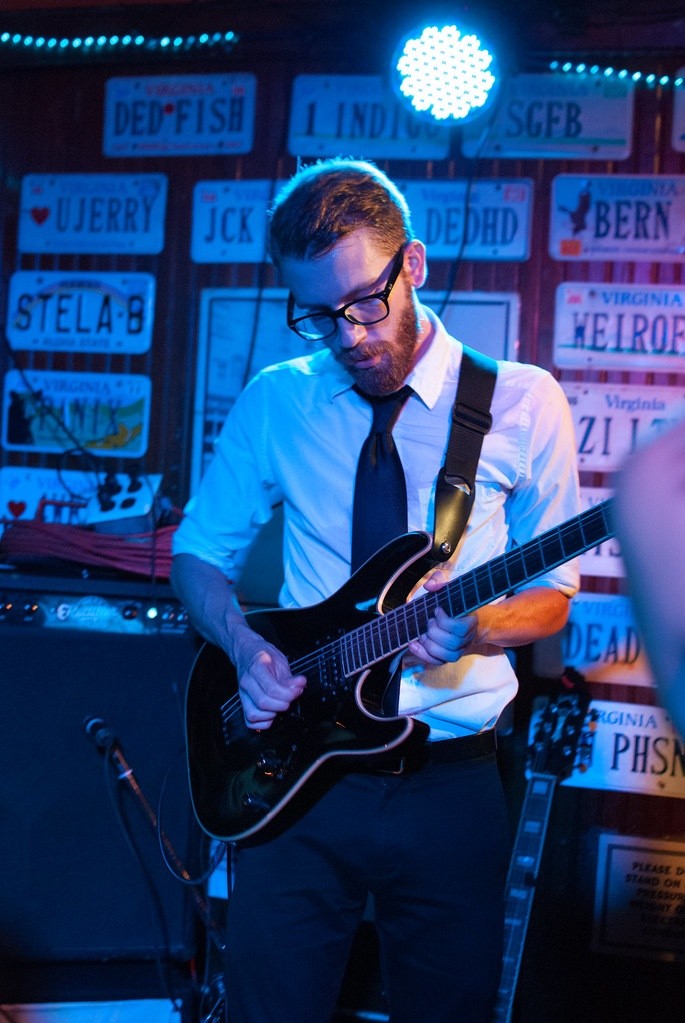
[326,732,497,774]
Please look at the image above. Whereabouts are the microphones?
[80,713,140,794]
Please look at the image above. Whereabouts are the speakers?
[0,569,208,969]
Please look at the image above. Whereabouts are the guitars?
[181,497,621,848]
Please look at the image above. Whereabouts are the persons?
[168,159,580,1023]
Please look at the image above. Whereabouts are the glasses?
[285,246,406,341]
